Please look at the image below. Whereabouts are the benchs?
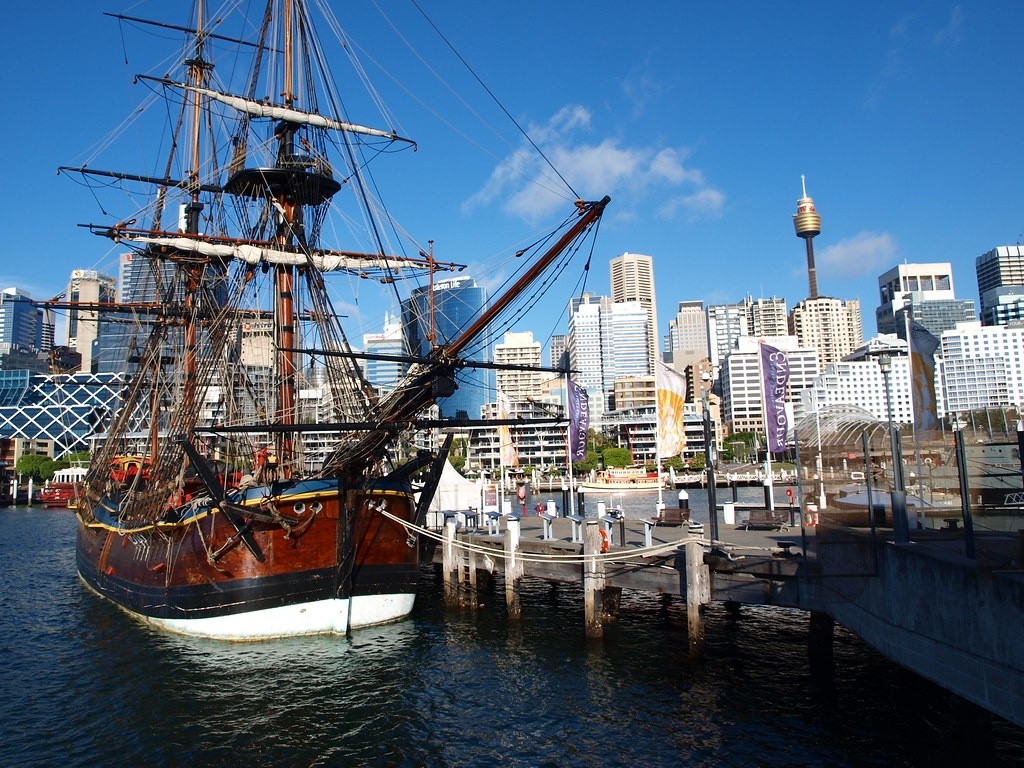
[653,509,691,529]
[743,510,791,533]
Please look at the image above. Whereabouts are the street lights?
[698,371,721,543]
[526,396,575,516]
[878,352,910,543]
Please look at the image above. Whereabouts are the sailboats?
[34,1,613,646]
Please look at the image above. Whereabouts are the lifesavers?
[598,528,609,554]
[803,508,817,527]
